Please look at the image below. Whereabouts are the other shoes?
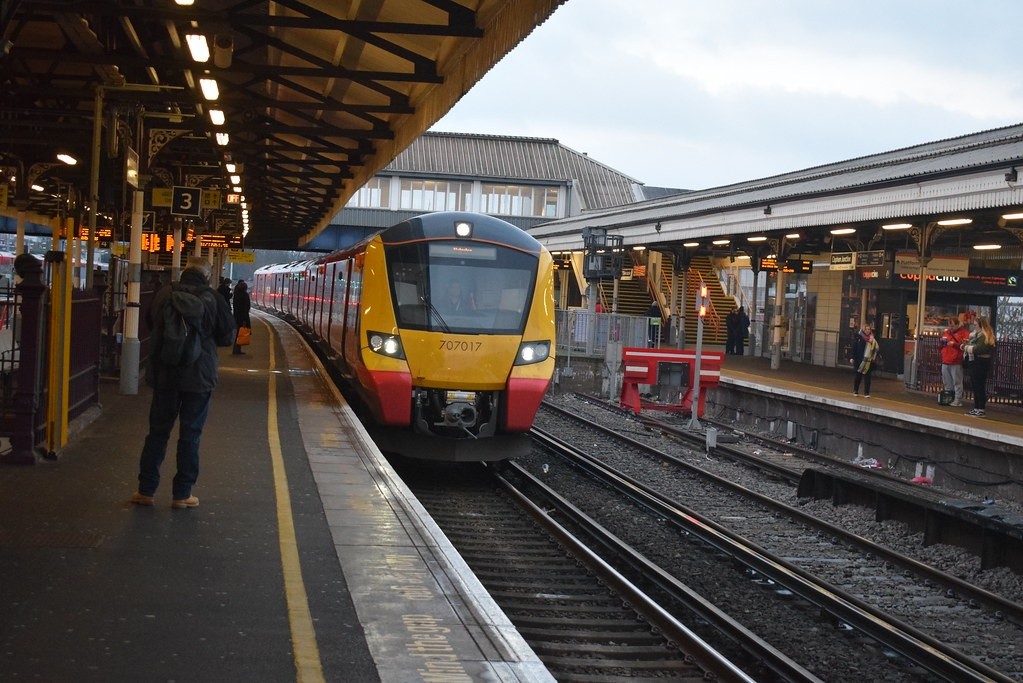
[853,392,858,395]
[863,394,869,397]
[949,402,961,406]
[232,351,245,355]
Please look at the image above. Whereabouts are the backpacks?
[148,279,202,367]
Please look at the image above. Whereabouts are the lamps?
[563,254,569,268]
[55,134,85,167]
[552,261,560,273]
[210,32,235,69]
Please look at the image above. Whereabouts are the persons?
[938,317,996,418]
[435,277,479,316]
[133,256,254,507]
[595,296,750,356]
[848,324,880,398]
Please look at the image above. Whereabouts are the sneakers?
[972,410,985,417]
[963,409,976,415]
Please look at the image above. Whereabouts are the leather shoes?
[172,494,199,508]
[132,491,153,506]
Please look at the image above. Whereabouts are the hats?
[237,282,244,288]
[224,278,231,283]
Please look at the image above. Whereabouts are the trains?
[250,211,557,460]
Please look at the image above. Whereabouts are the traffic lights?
[696,282,710,318]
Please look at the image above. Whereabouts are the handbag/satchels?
[236,326,252,344]
